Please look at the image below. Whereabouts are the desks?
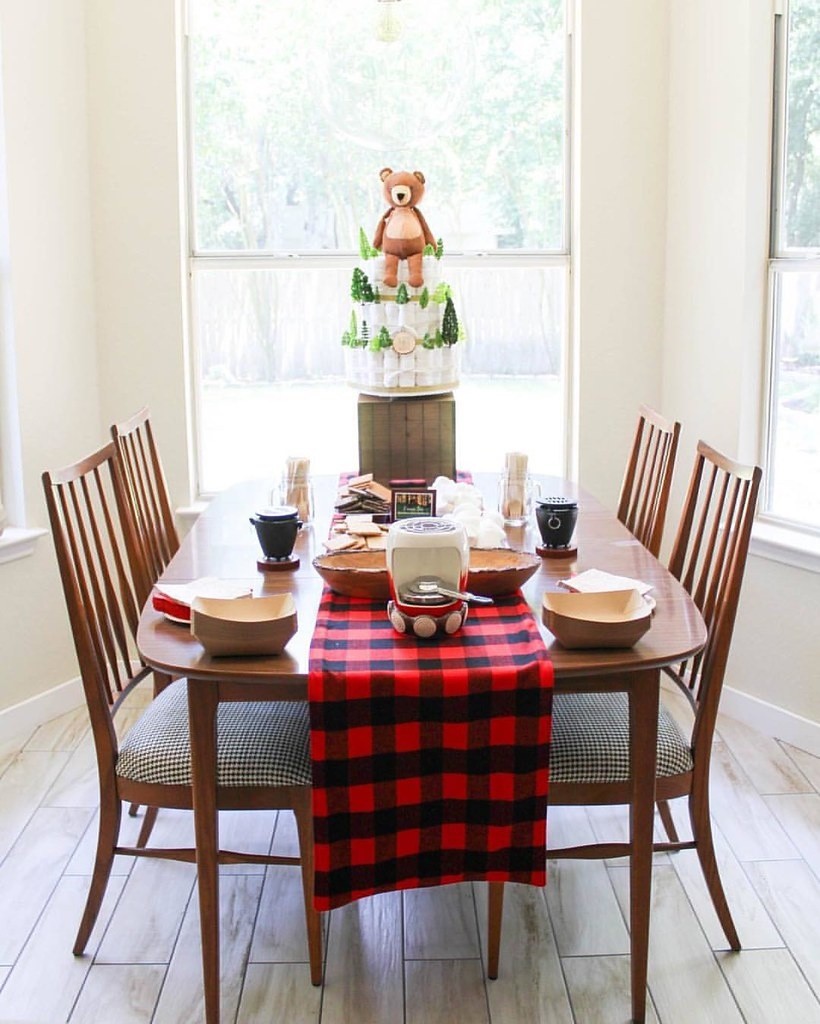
[137,472,708,1024]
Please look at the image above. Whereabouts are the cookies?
[321,520,391,552]
[330,473,394,513]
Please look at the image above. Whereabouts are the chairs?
[615,401,683,854]
[110,404,183,816]
[43,441,324,987]
[488,439,763,981]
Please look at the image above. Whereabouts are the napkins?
[557,568,654,597]
[153,576,252,604]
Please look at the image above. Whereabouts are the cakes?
[342,168,467,388]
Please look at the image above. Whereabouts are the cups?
[270,475,315,537]
[495,471,542,527]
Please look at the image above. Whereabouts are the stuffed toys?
[373,167,438,287]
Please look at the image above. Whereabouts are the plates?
[643,595,657,610]
[190,592,297,656]
[312,547,543,598]
[542,589,651,649]
[163,612,190,623]
